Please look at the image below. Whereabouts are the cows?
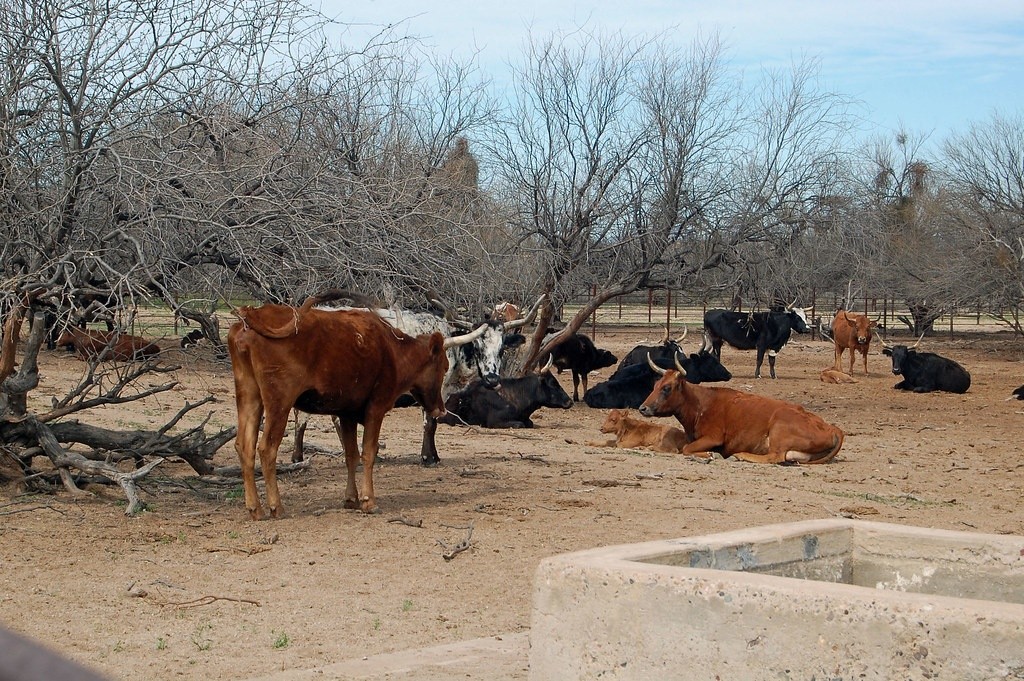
[1011,384,1024,401]
[819,301,970,396]
[226,289,490,521]
[290,289,845,465]
[180,329,204,350]
[0,275,161,363]
